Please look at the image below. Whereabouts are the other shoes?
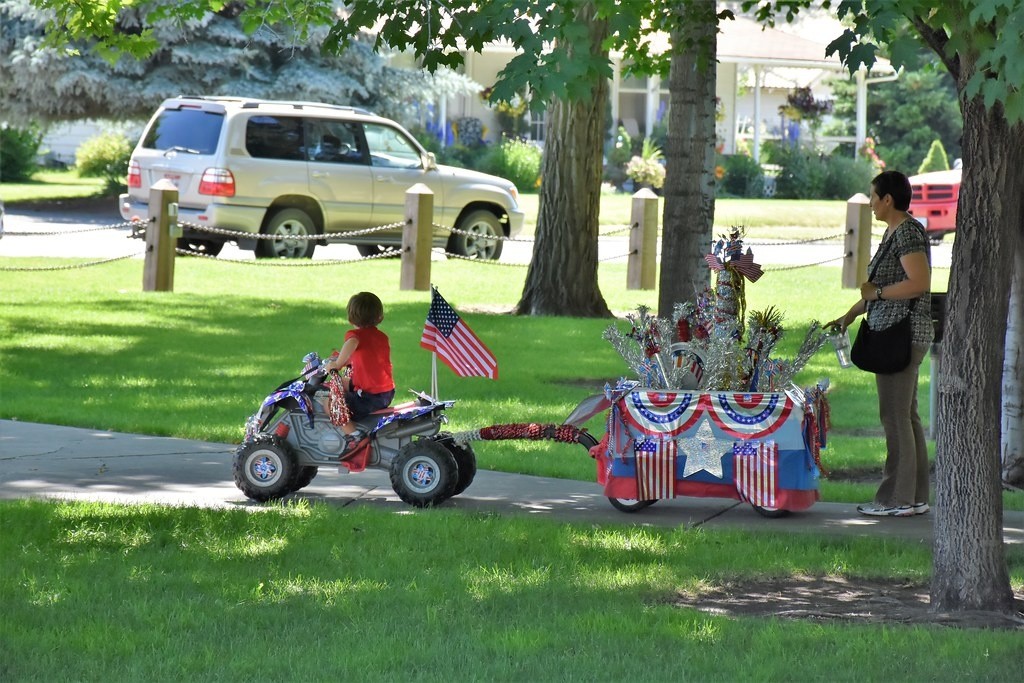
[338,429,370,462]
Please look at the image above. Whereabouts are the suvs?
[118,95,523,264]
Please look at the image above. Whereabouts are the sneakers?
[913,502,930,514]
[857,500,915,516]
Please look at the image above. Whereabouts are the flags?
[420,288,499,380]
[704,247,765,283]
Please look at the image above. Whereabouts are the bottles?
[829,324,854,368]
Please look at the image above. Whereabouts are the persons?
[323,291,395,460]
[821,171,935,515]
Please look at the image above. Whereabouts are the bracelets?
[876,288,885,302]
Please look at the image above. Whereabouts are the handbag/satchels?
[850,318,913,374]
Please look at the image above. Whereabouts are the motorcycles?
[232,365,476,510]
